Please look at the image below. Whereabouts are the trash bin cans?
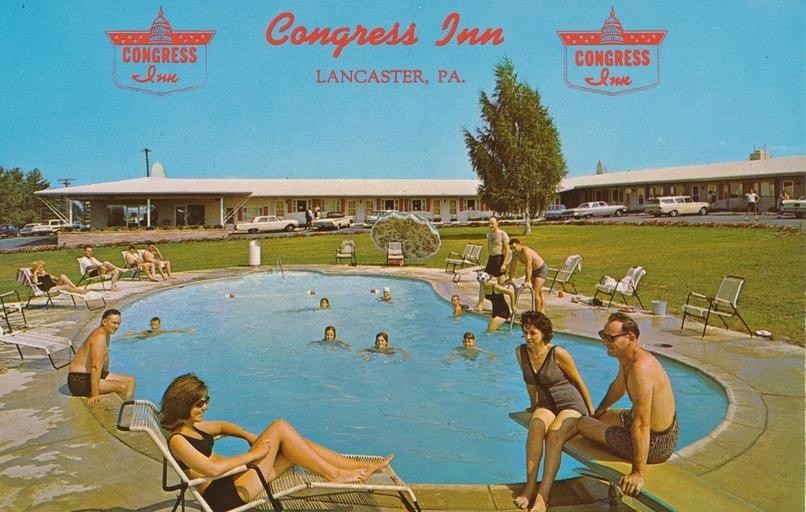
[248,240,260,267]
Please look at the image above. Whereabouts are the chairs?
[17,266,107,311]
[117,251,146,279]
[74,257,113,291]
[336,239,358,266]
[385,240,408,267]
[138,249,168,268]
[543,256,583,294]
[1,290,28,330]
[103,260,144,281]
[445,242,474,272]
[453,244,482,272]
[679,272,754,337]
[117,399,423,510]
[589,265,650,311]
[1,326,75,369]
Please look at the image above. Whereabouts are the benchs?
[509,410,754,511]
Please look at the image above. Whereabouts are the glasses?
[599,330,631,342]
[194,396,210,407]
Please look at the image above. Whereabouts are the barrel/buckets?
[651,301,666,314]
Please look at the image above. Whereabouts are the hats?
[478,272,490,282]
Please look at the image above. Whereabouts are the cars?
[232,215,300,234]
[363,209,396,227]
[562,200,629,220]
[546,204,570,220]
[0,219,89,238]
[645,195,711,217]
[781,195,806,218]
[310,210,355,231]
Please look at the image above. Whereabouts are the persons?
[575,309,680,499]
[305,203,322,232]
[778,191,791,216]
[509,309,595,512]
[154,372,394,511]
[464,215,511,315]
[27,237,548,370]
[67,308,136,404]
[743,188,760,220]
